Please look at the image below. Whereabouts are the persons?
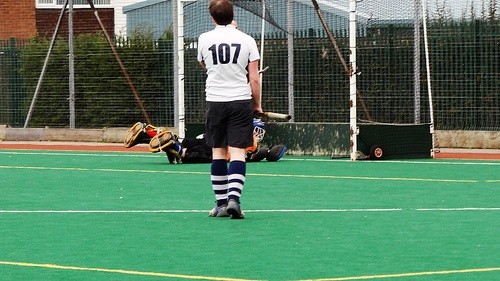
[124,116,271,165]
[195,0,265,222]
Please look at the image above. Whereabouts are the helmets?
[252,118,266,141]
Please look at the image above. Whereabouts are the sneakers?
[124,122,146,148]
[209,203,229,217]
[227,199,244,218]
[149,130,175,152]
